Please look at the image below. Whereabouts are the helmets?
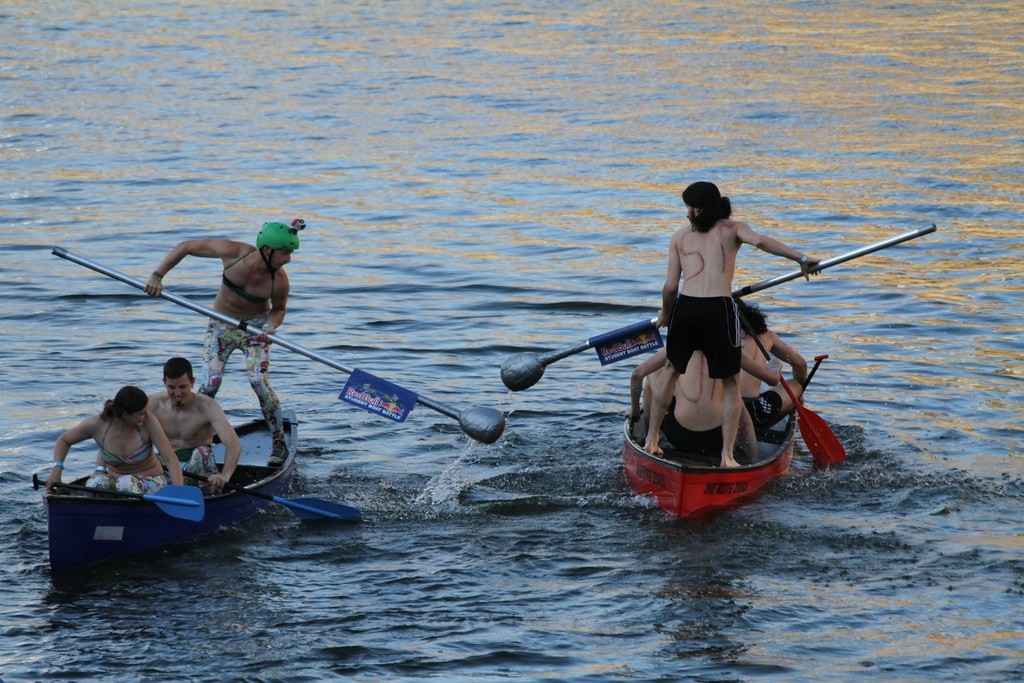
[256,221,299,251]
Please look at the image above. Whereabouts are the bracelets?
[53,461,64,469]
[262,323,273,329]
[151,271,164,281]
[96,466,107,473]
[798,254,808,264]
[768,365,779,372]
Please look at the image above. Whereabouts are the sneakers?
[267,441,289,465]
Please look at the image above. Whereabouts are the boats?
[44,409,298,569]
[622,404,797,514]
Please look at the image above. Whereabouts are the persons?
[143,218,307,466]
[144,355,242,496]
[641,181,822,468]
[44,385,185,499]
[631,298,809,454]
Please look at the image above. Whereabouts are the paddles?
[162,464,362,521]
[33,473,205,521]
[738,307,846,465]
[800,355,829,391]
